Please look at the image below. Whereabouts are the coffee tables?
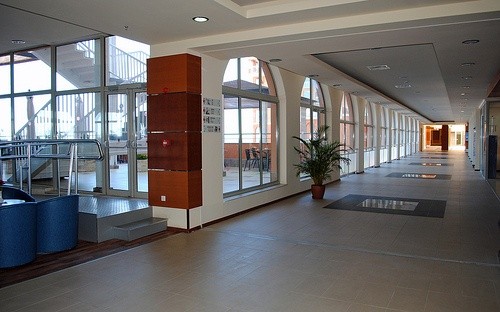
[0,198,26,206]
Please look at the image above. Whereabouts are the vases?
[0,190,4,205]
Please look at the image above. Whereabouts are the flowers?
[0,179,5,186]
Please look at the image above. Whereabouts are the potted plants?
[291,124,355,200]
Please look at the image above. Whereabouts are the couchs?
[0,185,35,202]
[0,201,37,267]
[35,194,80,254]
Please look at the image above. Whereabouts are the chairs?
[250,147,264,170]
[244,149,260,172]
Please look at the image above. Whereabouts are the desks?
[257,147,271,172]
